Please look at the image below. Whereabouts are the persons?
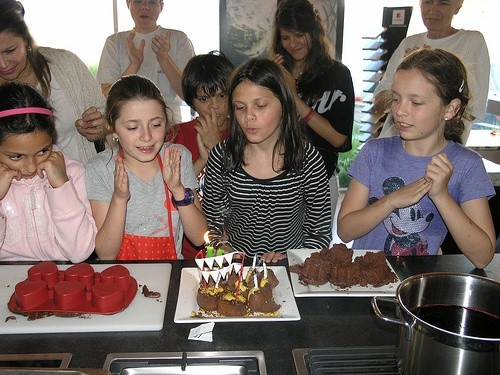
[203,59,331,265]
[262,0,355,221]
[372,0,491,146]
[0,0,110,165]
[336,49,496,269]
[83,74,210,259]
[94,0,196,124]
[0,81,98,263]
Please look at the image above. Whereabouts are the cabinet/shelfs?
[356,25,387,151]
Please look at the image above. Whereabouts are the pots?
[371,271,500,375]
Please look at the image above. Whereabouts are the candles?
[203,231,214,257]
[250,254,268,287]
[215,272,222,288]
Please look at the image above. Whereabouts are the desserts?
[298,243,391,288]
[196,266,281,317]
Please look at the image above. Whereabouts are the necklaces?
[295,61,304,74]
[15,71,32,84]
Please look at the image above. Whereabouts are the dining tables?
[0,251,500,375]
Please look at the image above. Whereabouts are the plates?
[0,263,173,334]
[286,249,403,298]
[174,264,301,323]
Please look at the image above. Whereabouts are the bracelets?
[301,107,315,123]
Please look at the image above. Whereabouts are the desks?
[466,129,500,151]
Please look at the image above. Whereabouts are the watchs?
[171,188,195,207]
